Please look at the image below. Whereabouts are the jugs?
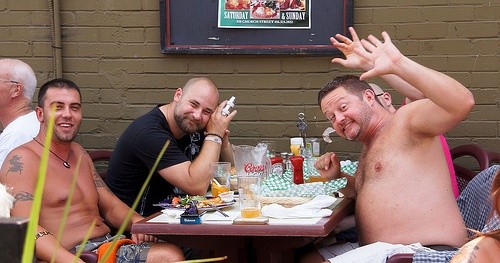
[229,145,272,188]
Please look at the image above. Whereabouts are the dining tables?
[132,198,355,263]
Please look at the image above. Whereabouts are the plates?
[224,0,306,20]
[157,198,235,211]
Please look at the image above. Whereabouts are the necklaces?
[33,137,72,168]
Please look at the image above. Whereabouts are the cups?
[208,162,232,196]
[260,141,278,158]
[236,175,262,217]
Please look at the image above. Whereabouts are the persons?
[0,58,49,223]
[106,73,252,263]
[0,77,187,263]
[304,27,499,263]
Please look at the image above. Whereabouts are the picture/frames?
[157,0,355,57]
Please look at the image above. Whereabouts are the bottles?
[290,156,305,184]
[271,157,284,176]
[289,137,304,157]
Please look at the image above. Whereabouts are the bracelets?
[205,138,222,145]
[34,230,54,239]
[204,131,224,141]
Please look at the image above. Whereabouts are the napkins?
[261,193,336,220]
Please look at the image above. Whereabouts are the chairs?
[385,144,500,263]
